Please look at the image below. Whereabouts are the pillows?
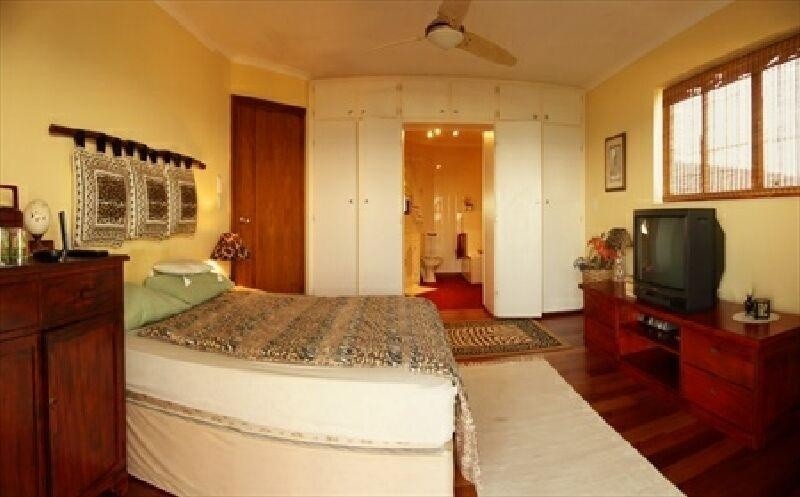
[124,263,235,333]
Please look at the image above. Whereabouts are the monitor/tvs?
[632,209,726,313]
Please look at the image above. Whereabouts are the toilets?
[420,236,443,283]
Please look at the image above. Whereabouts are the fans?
[365,1,518,65]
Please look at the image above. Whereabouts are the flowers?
[574,234,619,271]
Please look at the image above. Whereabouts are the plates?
[731,311,781,324]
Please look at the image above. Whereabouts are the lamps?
[211,232,254,283]
[604,228,633,282]
[426,25,464,50]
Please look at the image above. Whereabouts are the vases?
[581,268,614,283]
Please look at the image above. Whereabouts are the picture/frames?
[604,134,626,192]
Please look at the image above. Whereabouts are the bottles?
[743,295,771,320]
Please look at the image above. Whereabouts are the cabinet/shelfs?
[0,260,125,497]
[125,289,457,496]
[310,122,405,297]
[313,78,584,123]
[579,286,800,456]
[484,122,586,320]
